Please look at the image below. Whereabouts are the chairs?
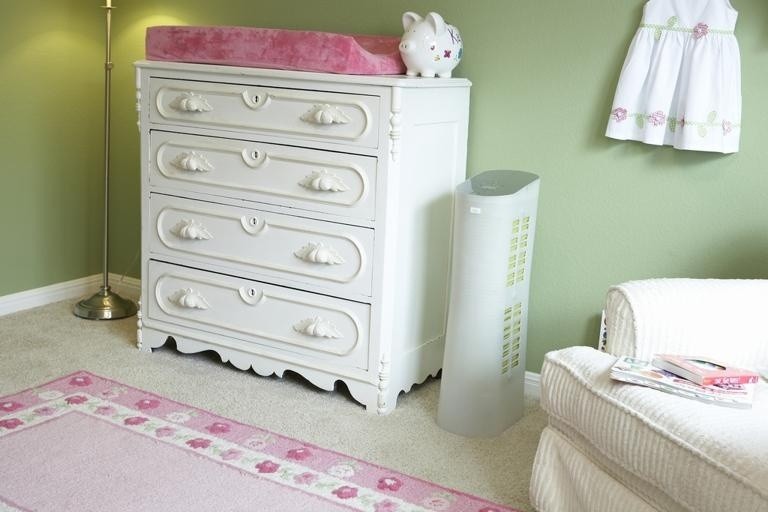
[525,279,768,512]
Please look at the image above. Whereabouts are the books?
[609,353,760,410]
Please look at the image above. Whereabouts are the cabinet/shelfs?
[135,60,473,415]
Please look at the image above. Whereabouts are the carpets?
[0,369,526,511]
